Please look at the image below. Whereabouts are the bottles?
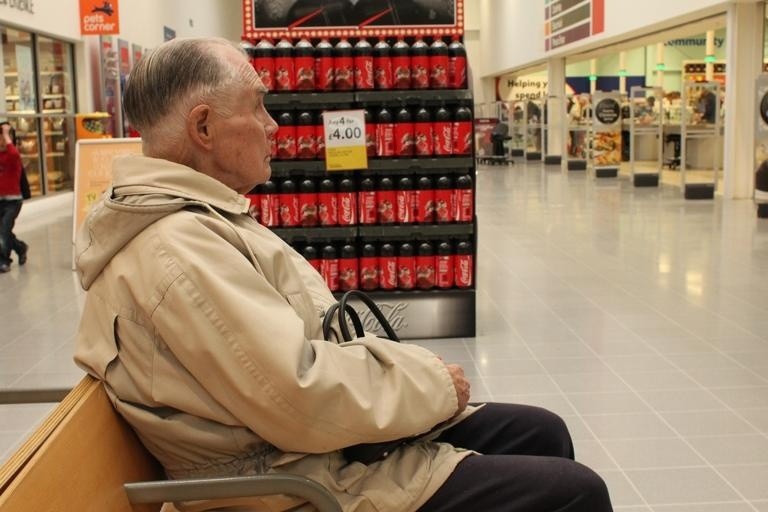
[269,101,474,159]
[238,34,468,94]
[301,239,473,292]
[245,173,474,228]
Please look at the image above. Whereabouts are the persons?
[1,121,32,274]
[70,36,615,512]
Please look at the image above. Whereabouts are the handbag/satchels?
[321,289,400,467]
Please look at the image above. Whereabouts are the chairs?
[0,371,343,511]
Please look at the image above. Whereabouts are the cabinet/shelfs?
[237,34,476,339]
[6,71,70,191]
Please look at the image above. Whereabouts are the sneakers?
[18,241,28,266]
[1,263,11,274]
